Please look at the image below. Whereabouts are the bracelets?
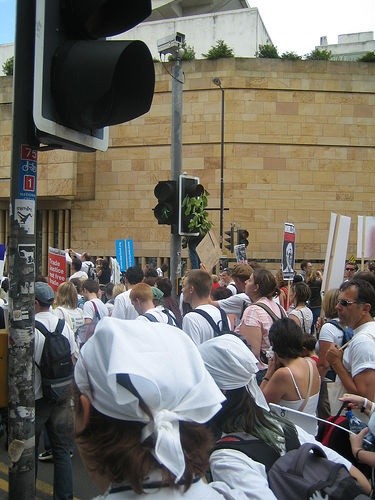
[355,448,365,463]
[261,376,270,381]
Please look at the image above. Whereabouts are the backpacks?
[143,312,174,327]
[324,320,354,383]
[209,417,374,499]
[1,298,9,329]
[32,319,75,405]
[190,307,229,335]
[240,301,287,366]
[85,262,96,279]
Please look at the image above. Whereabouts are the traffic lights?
[224,231,233,253]
[237,229,248,248]
[178,175,203,236]
[153,180,177,225]
[33,1,155,153]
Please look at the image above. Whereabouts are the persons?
[206,263,255,332]
[156,261,168,278]
[143,264,158,287]
[53,278,85,335]
[239,268,289,368]
[342,260,358,278]
[73,316,246,500]
[283,277,313,338]
[106,282,125,307]
[183,270,232,347]
[299,259,314,278]
[218,266,238,298]
[152,276,183,329]
[194,328,371,500]
[64,245,110,292]
[111,266,145,320]
[29,273,78,500]
[336,385,375,467]
[129,281,178,327]
[80,277,108,346]
[324,278,375,400]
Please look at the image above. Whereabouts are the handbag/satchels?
[296,357,313,411]
[230,283,238,294]
[91,300,101,320]
[314,401,374,480]
[298,310,305,333]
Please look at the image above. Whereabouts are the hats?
[226,264,254,280]
[35,281,55,305]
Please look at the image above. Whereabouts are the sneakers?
[38,450,54,460]
[70,450,75,458]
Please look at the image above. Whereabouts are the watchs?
[360,397,369,414]
[258,311,324,444]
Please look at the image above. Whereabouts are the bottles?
[345,411,375,451]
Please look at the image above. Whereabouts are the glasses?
[336,300,364,307]
[223,268,230,273]
